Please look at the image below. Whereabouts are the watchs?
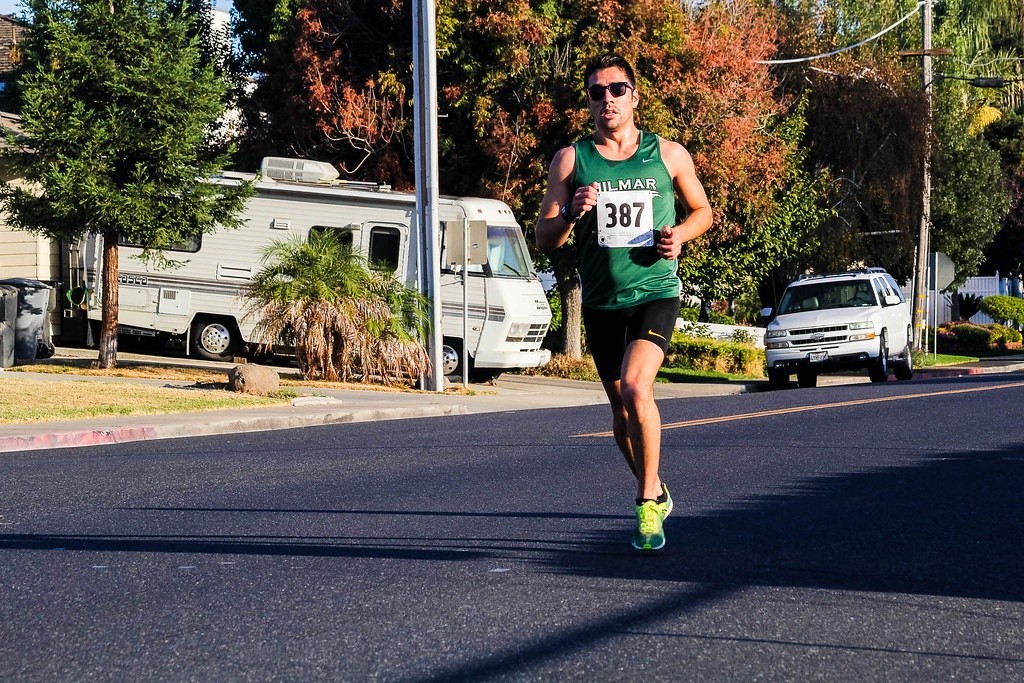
[561,201,582,223]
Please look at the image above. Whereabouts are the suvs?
[762,265,924,385]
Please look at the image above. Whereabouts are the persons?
[535,51,713,550]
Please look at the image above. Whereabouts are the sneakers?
[632,501,665,549]
[656,482,674,518]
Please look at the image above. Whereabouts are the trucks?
[82,157,556,380]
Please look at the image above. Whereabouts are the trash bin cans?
[0,277,64,368]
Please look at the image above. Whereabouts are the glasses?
[587,81,634,102]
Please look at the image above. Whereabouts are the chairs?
[823,289,842,306]
[802,297,819,308]
[855,291,871,302]
